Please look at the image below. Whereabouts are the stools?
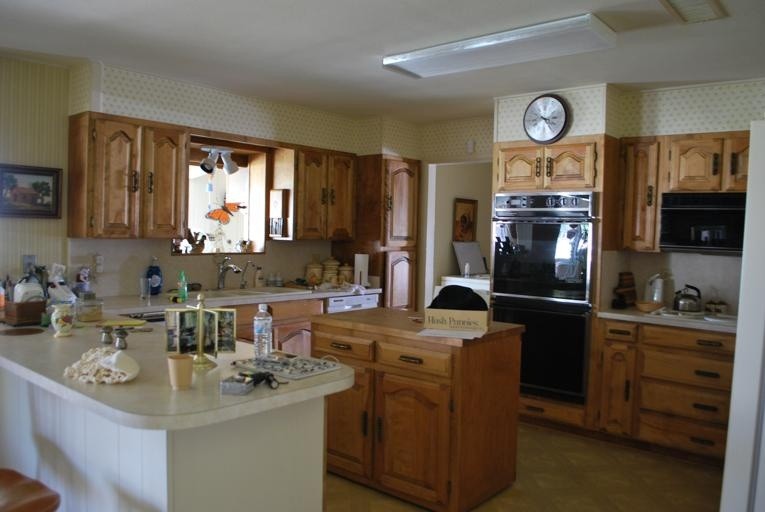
[0,467,60,512]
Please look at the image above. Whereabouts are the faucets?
[240,261,255,287]
[685,284,701,300]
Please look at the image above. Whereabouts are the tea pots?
[674,284,703,312]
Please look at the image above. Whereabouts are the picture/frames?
[0,163,63,218]
[453,198,478,242]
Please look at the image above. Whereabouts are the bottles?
[49,303,74,338]
[255,266,284,287]
[305,255,354,286]
[464,262,470,278]
[253,302,273,360]
[74,291,103,321]
[99,326,127,351]
[177,270,188,301]
[145,256,163,296]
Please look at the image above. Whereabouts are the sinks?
[217,256,243,290]
[188,288,313,301]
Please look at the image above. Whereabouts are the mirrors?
[171,140,266,256]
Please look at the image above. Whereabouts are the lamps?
[200,147,239,175]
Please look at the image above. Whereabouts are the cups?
[139,277,152,303]
[166,353,194,392]
[705,304,727,314]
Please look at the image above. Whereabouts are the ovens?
[491,191,592,406]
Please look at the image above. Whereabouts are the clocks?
[523,94,572,144]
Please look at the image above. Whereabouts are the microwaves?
[658,192,745,255]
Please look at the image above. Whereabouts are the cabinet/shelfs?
[269,141,357,243]
[664,130,750,193]
[634,324,736,459]
[363,249,416,313]
[66,111,184,239]
[618,136,664,253]
[493,135,598,192]
[229,300,323,359]
[585,317,639,441]
[353,154,421,247]
[310,307,525,512]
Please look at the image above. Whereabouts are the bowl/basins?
[635,300,663,313]
